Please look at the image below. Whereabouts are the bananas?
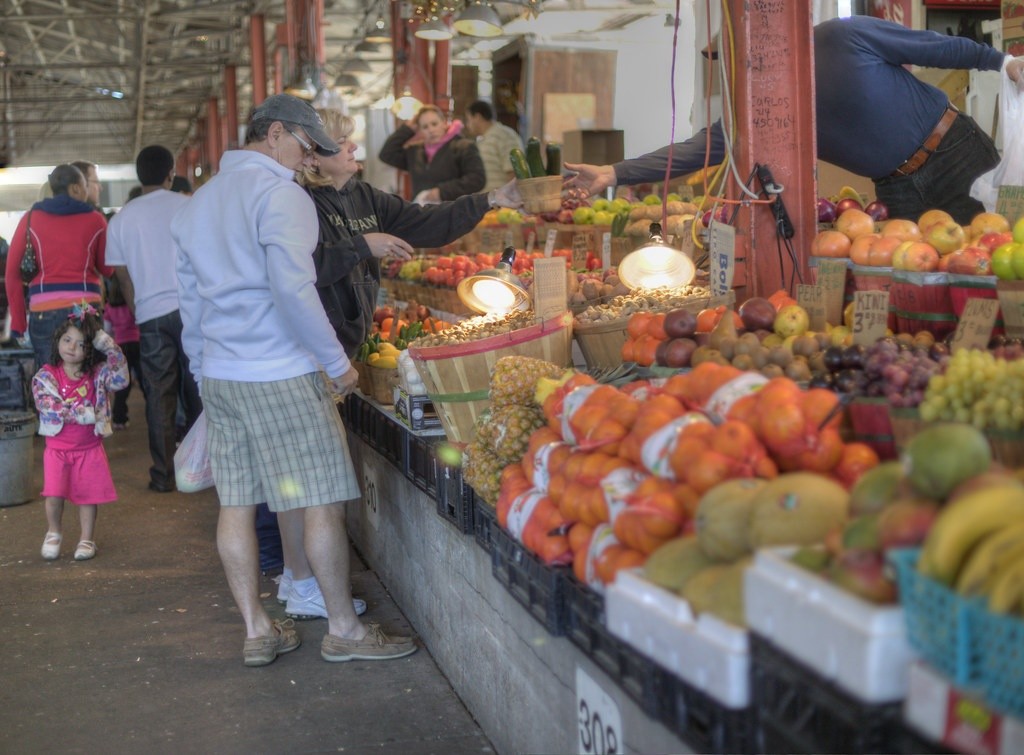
[917,480,1024,625]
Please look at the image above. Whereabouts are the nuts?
[411,308,539,348]
[572,285,710,323]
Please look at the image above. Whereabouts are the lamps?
[393,85,423,120]
[333,27,392,87]
[455,244,534,317]
[617,221,695,290]
[285,66,318,100]
[412,0,503,40]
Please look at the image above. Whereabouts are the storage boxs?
[338,388,1023,755]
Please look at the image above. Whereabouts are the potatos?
[622,202,706,237]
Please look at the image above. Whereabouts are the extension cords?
[757,165,795,239]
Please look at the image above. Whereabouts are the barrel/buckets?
[811,258,1023,351]
[465,176,709,267]
[800,382,1024,470]
[573,287,736,374]
[407,312,574,446]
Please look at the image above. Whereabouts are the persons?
[463,100,523,193]
[0,237,9,337]
[562,15,1024,225]
[277,107,524,619]
[71,159,117,221]
[171,93,417,668]
[378,103,486,202]
[4,164,118,374]
[104,145,203,494]
[31,296,131,560]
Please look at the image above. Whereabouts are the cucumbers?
[510,136,562,180]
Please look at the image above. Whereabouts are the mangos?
[367,341,402,369]
[791,418,1024,607]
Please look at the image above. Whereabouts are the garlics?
[396,348,429,395]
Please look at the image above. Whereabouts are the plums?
[809,331,1024,394]
[703,197,886,225]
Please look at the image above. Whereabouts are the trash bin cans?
[0,411,36,507]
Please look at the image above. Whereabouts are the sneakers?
[276,571,303,603]
[284,586,367,619]
[321,623,418,662]
[242,618,301,666]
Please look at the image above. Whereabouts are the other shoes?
[111,419,130,430]
[149,482,170,493]
[74,540,98,560]
[41,531,63,559]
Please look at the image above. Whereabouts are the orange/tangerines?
[375,317,451,341]
[498,360,876,585]
[623,287,801,367]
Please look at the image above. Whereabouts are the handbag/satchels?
[21,210,39,283]
[990,54,1024,188]
[172,406,217,492]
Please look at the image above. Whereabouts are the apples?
[658,298,858,362]
[810,209,1024,281]
[478,194,711,226]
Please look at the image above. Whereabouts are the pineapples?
[462,353,640,509]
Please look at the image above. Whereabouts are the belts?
[890,101,961,178]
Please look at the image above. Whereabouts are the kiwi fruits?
[717,331,934,380]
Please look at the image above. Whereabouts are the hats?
[251,93,342,157]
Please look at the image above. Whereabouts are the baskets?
[887,549,1024,715]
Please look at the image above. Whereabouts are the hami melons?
[638,475,850,625]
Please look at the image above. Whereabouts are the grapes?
[878,343,1024,433]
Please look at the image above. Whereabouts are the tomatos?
[386,248,604,289]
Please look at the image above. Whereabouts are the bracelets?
[491,186,501,211]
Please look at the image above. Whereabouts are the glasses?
[281,123,315,155]
